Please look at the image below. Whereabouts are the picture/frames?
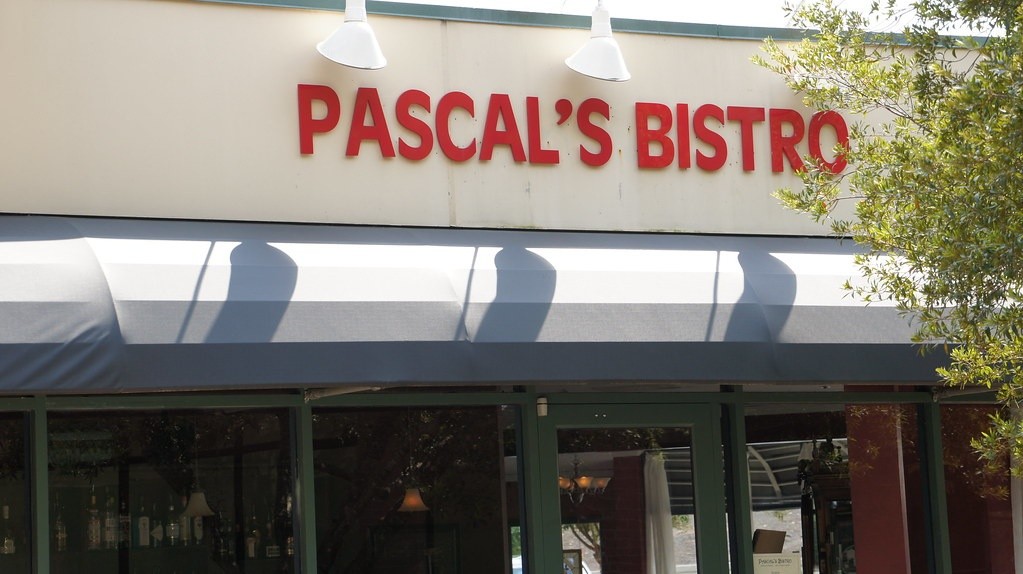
[562,549,583,574]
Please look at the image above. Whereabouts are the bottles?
[102,496,118,553]
[247,515,260,560]
[132,496,150,548]
[151,502,164,549]
[85,485,101,553]
[53,505,66,552]
[283,518,294,559]
[165,504,178,547]
[179,496,192,547]
[0,498,16,555]
[260,520,281,560]
[118,498,132,550]
[211,511,243,561]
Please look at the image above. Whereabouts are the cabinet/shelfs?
[810,472,858,574]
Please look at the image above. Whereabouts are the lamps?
[316,0,388,70]
[537,397,548,417]
[397,408,431,514]
[179,414,216,518]
[565,0,639,82]
[558,453,611,506]
